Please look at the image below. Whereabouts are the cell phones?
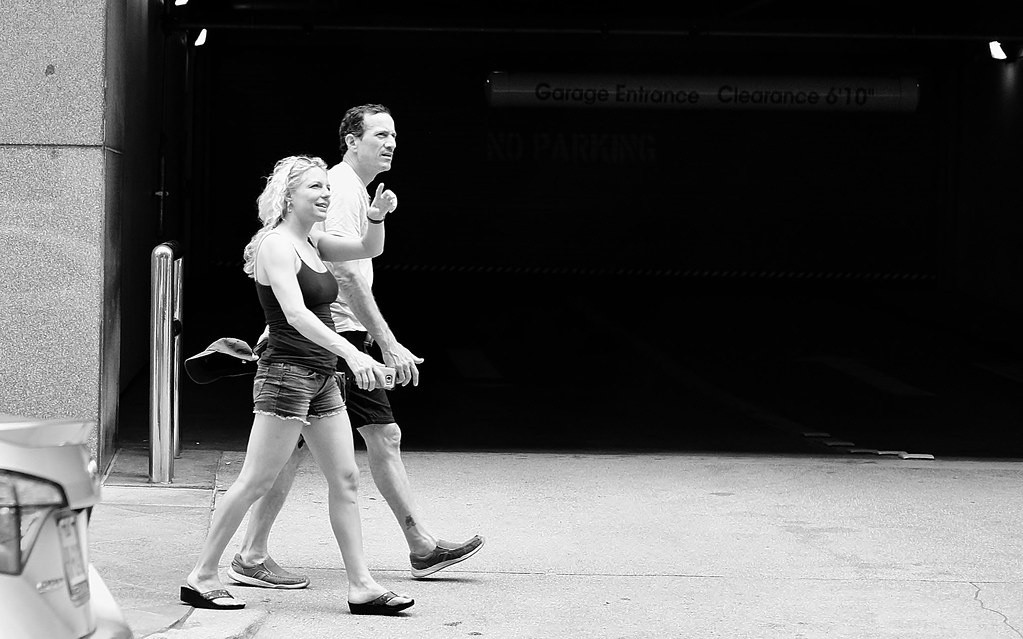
[372,366,396,391]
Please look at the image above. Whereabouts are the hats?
[183,337,260,386]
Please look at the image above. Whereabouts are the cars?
[1,412,133,639]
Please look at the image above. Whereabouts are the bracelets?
[367,214,384,224]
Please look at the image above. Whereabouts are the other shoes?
[227,552,310,588]
[409,535,484,577]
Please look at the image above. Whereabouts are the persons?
[180,155,414,614]
[228,103,485,589]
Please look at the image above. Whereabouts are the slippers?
[180,581,246,610]
[347,591,414,614]
